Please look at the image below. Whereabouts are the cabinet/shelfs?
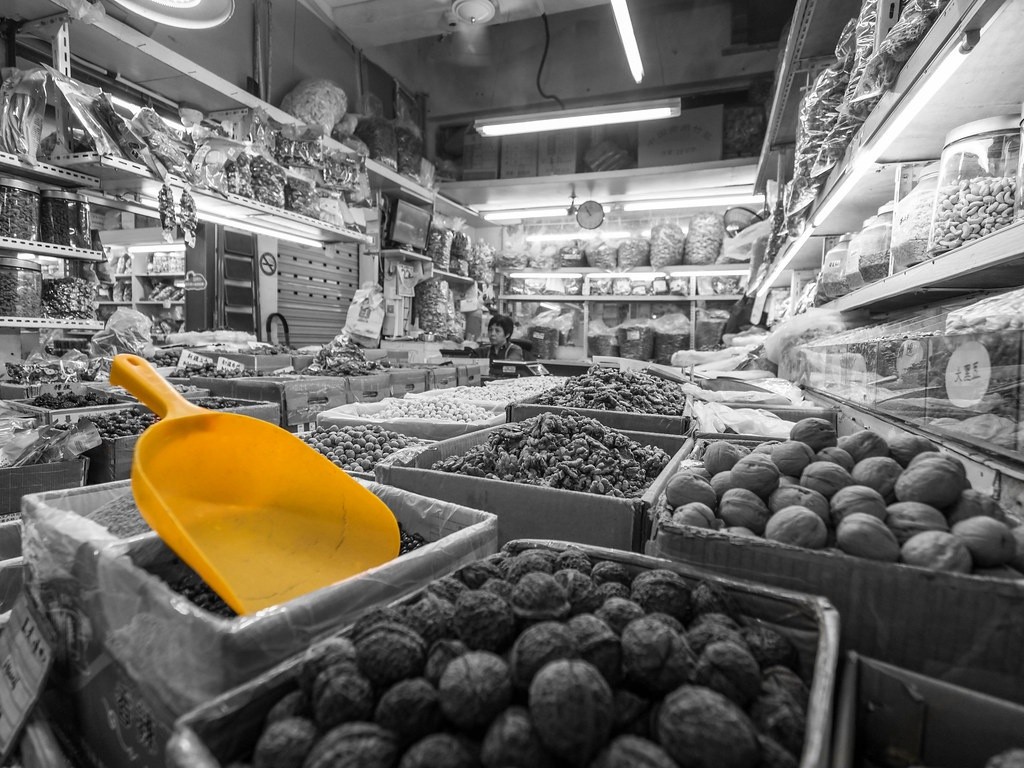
[0,0,1024,482]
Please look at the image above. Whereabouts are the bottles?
[815,111,1024,303]
[0,178,42,241]
[40,189,92,250]
[0,257,43,319]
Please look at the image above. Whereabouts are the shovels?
[107,349,400,617]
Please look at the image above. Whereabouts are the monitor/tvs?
[384,196,433,251]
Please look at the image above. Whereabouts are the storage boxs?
[0,285,1024,768]
[539,127,591,176]
[462,132,501,180]
[499,133,538,179]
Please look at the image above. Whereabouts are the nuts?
[664,418,1024,575]
[932,171,1017,254]
[432,362,686,501]
[250,545,811,768]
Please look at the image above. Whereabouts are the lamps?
[134,193,325,248]
[473,10,682,138]
[114,0,235,30]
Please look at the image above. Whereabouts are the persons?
[471,314,523,386]
